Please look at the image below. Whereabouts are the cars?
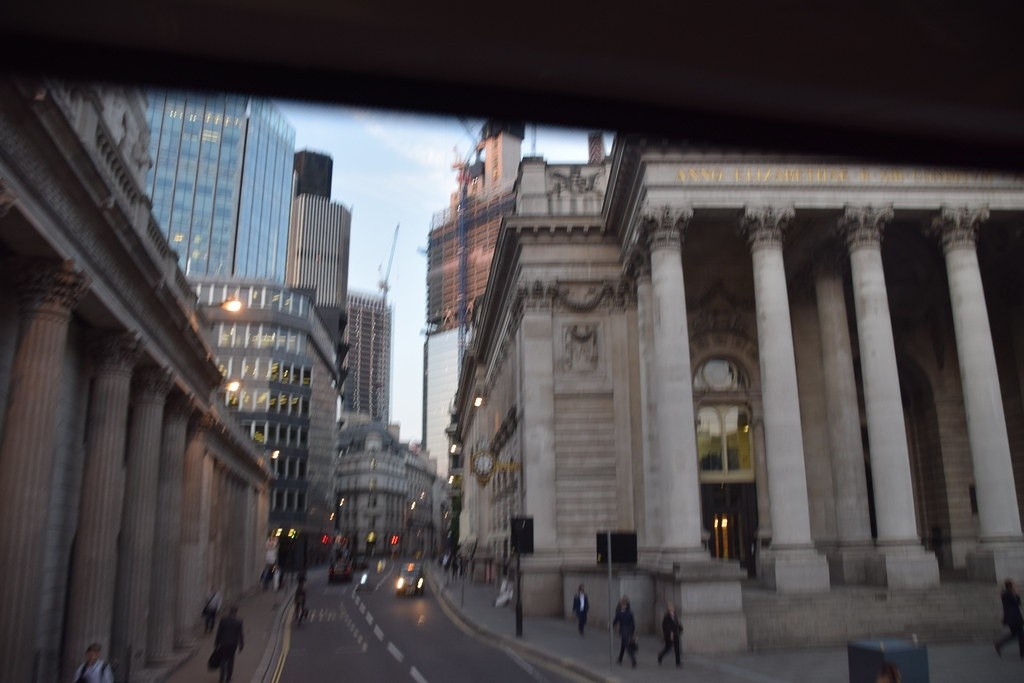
[351,554,369,569]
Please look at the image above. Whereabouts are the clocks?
[200,297,244,313]
[471,450,497,476]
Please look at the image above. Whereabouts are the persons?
[657,600,685,669]
[609,600,639,669]
[212,604,246,683]
[571,583,589,636]
[992,578,1024,660]
[202,583,223,633]
[73,644,115,683]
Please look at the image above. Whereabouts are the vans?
[398,563,423,596]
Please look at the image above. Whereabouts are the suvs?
[329,560,353,583]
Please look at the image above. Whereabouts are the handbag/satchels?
[202,607,207,615]
[208,649,220,668]
[629,636,638,651]
